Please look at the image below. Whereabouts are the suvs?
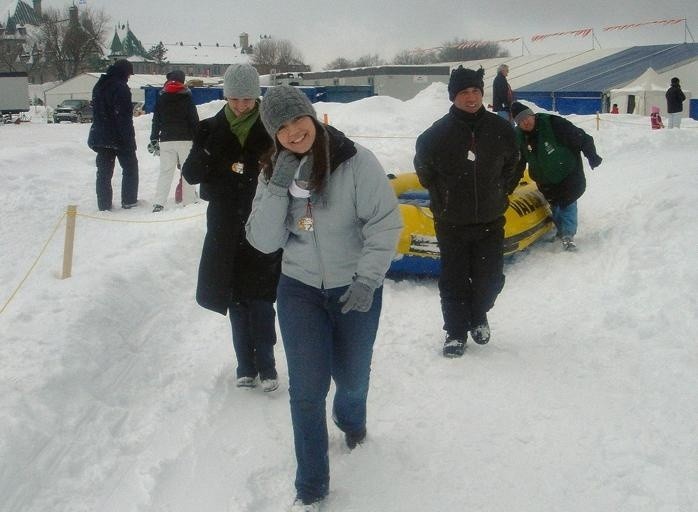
[53,98,93,123]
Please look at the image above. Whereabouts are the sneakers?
[289,495,327,512]
[342,433,368,451]
[236,373,257,388]
[442,333,469,358]
[562,237,577,253]
[259,370,280,392]
[471,316,491,345]
[122,200,139,209]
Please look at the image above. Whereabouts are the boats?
[387,168,553,276]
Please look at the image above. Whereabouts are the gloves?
[588,152,603,171]
[339,281,375,314]
[269,148,300,189]
[213,133,241,172]
[148,140,160,157]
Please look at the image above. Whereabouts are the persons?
[413,66,521,358]
[87,60,144,211]
[665,77,685,130]
[648,104,664,132]
[147,68,203,211]
[492,63,516,123]
[510,100,603,251]
[243,84,405,511]
[182,63,281,392]
[611,103,620,115]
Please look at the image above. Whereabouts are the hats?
[113,58,136,75]
[166,70,186,84]
[650,106,660,113]
[258,81,332,185]
[223,63,262,101]
[511,101,535,124]
[447,63,487,101]
